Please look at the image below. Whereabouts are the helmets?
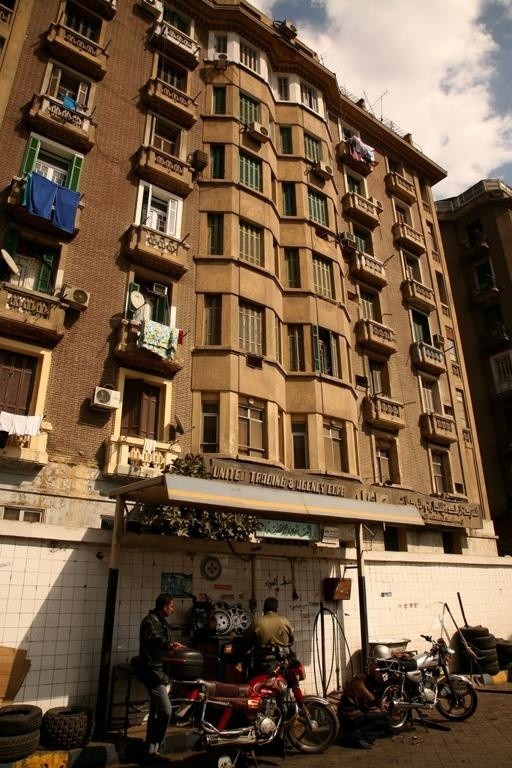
[372,644,392,660]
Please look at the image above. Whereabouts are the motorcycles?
[161,641,339,768]
[366,635,478,734]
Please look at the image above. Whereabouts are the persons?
[232,596,295,682]
[137,591,187,762]
[336,670,393,749]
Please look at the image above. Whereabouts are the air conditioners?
[316,161,333,181]
[341,231,355,242]
[280,19,297,40]
[61,283,90,311]
[433,333,445,346]
[214,52,229,64]
[249,121,270,144]
[192,149,208,171]
[90,387,121,412]
[367,196,384,214]
[151,283,167,295]
[142,0,163,18]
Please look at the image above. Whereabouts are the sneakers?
[146,742,160,755]
[352,729,372,749]
